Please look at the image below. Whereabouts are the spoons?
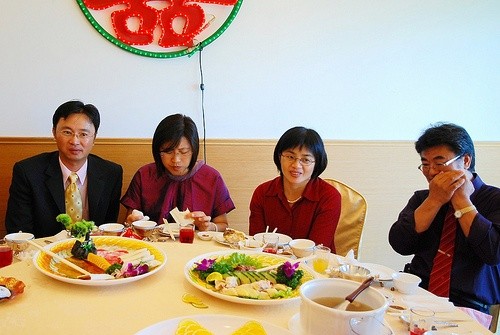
[399,315,459,328]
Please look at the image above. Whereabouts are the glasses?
[281,153,315,166]
[55,128,96,138]
[158,148,192,157]
[418,155,460,172]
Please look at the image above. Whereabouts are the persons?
[248,127,341,255]
[389,124,499,314]
[121,114,236,231]
[4,101,124,240]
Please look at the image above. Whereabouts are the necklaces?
[285,196,301,203]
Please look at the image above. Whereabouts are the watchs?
[454,205,477,218]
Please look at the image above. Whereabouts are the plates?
[254,233,292,246]
[139,315,293,335]
[184,250,319,305]
[197,231,214,241]
[158,222,195,237]
[354,262,396,281]
[213,234,249,246]
[0,276,25,304]
[33,236,168,286]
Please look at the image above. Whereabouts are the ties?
[427,207,458,301]
[65,173,83,226]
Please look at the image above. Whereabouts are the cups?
[179,226,194,243]
[0,240,13,269]
[262,234,279,254]
[409,306,435,335]
[313,245,331,273]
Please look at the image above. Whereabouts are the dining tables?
[0,229,498,335]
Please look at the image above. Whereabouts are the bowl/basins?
[289,239,315,258]
[340,264,371,282]
[5,233,34,247]
[99,223,124,237]
[133,220,157,241]
[392,272,422,294]
[299,278,393,335]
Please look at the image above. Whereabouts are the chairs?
[313,177,369,263]
